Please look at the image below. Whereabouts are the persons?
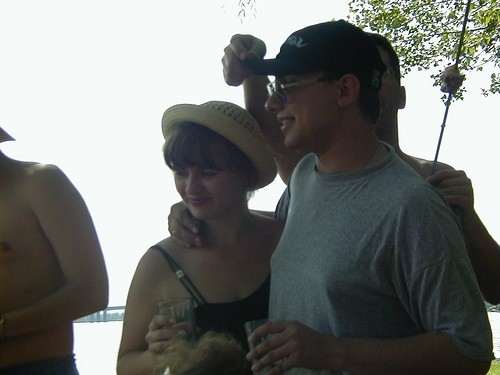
[223,31,500,305]
[116,100,284,375]
[0,128,109,375]
[167,19,493,375]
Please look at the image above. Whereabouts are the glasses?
[266,76,330,106]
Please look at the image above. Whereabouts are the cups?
[155,297,197,342]
[245,319,287,375]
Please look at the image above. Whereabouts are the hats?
[251,20,381,80]
[162,100,278,191]
